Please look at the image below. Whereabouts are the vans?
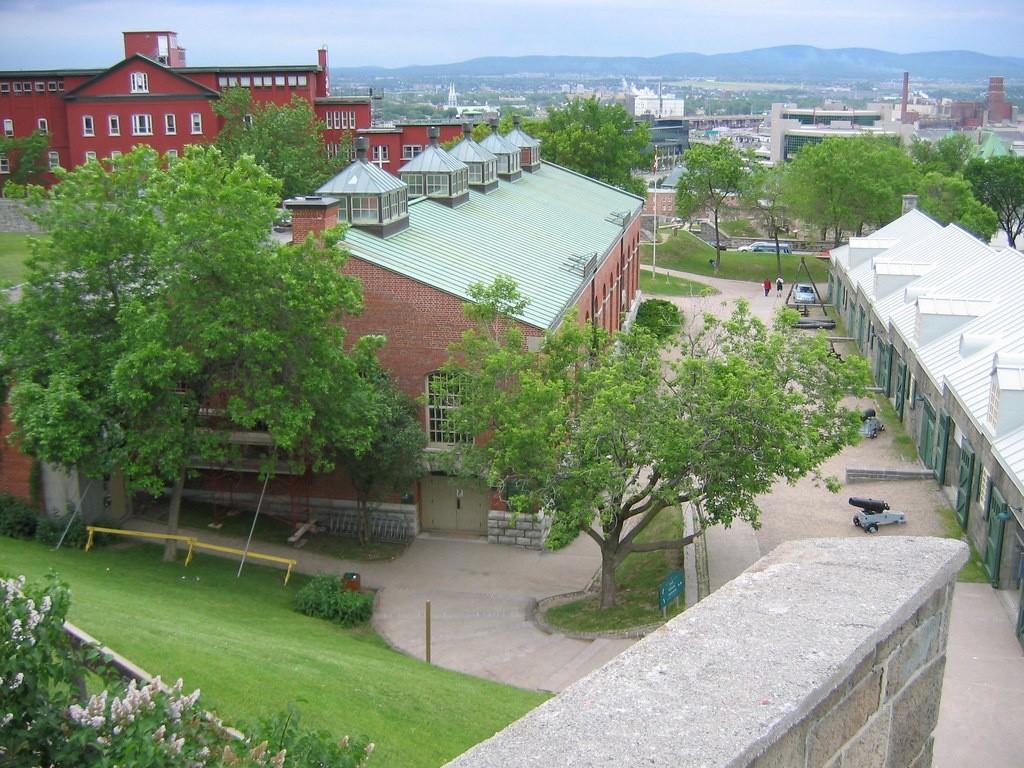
[750,241,792,255]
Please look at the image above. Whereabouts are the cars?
[793,284,815,304]
[737,242,769,252]
[709,241,727,251]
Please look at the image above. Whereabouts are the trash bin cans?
[342,572,361,592]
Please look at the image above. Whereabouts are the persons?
[709,259,717,267]
[764,276,784,298]
